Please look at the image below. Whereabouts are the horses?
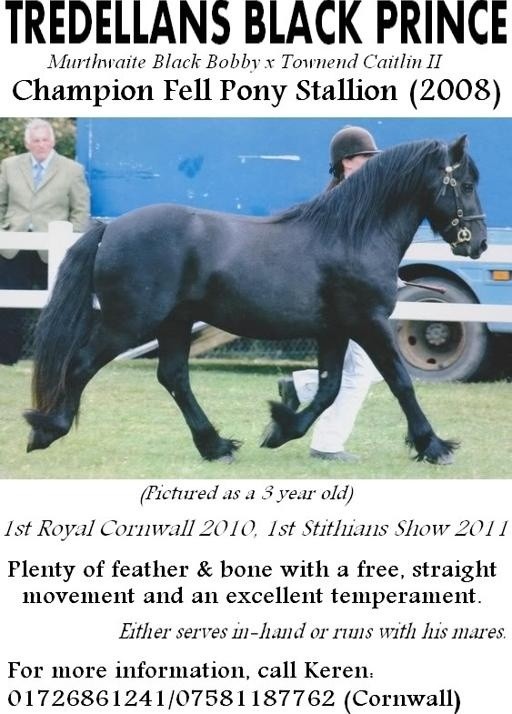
[21,136,488,467]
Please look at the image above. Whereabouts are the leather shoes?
[279,379,300,413]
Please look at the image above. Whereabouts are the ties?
[33,165,43,191]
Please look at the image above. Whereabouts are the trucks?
[74,118,512,382]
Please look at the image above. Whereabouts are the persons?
[279,127,405,463]
[0,120,89,365]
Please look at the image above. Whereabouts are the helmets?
[330,126,385,160]
